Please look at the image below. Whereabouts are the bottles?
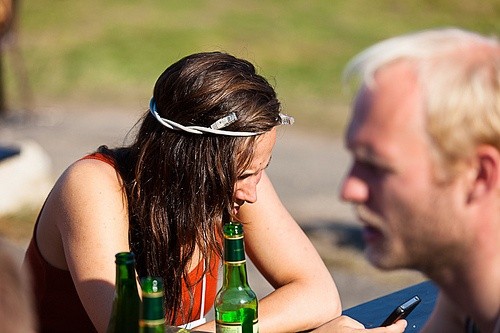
[214,221,259,333]
[106,252,142,333]
[141,276,167,333]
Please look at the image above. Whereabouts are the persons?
[338,27,500,333]
[23,51,407,333]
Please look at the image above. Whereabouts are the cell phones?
[378,295,422,327]
